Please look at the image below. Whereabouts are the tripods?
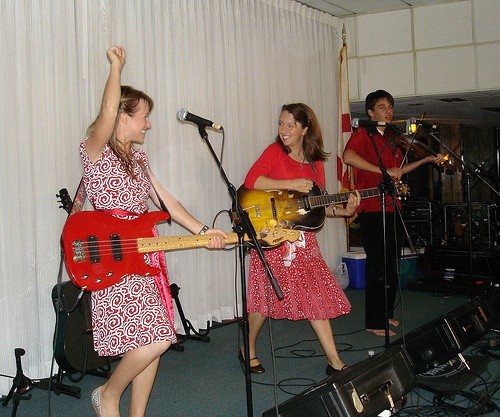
[3,348,57,417]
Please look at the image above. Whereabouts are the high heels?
[238,349,266,373]
[326,364,349,376]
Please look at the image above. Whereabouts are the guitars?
[51,187,109,375]
[58,208,300,291]
[230,181,412,249]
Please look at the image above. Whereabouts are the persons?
[79,43,229,417]
[343,89,445,338]
[236,101,350,377]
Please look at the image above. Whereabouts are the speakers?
[387,298,496,373]
[263,345,416,417]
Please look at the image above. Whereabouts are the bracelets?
[332,206,338,217]
[199,225,210,235]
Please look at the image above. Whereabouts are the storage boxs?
[343,253,418,289]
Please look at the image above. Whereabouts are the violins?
[389,130,461,171]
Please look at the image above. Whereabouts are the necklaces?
[293,155,305,169]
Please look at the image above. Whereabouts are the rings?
[308,187,310,190]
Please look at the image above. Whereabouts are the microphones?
[408,117,438,129]
[350,118,389,127]
[176,110,223,131]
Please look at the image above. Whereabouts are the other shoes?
[91,386,103,417]
